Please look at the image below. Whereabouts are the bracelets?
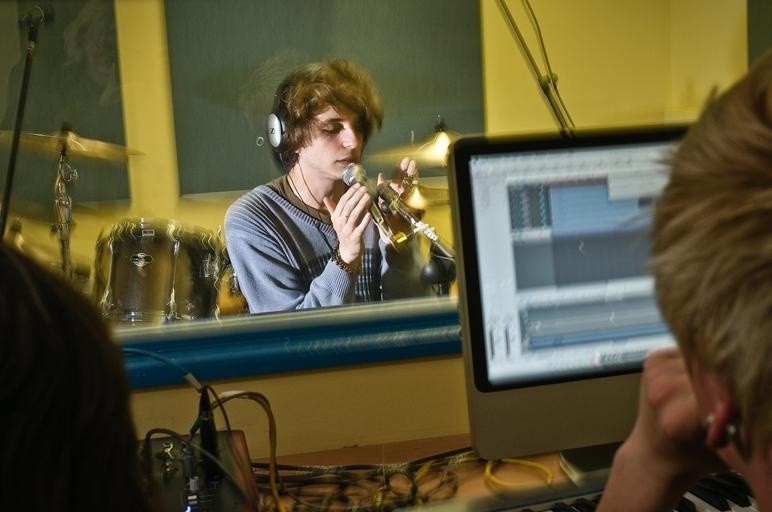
[330,242,363,274]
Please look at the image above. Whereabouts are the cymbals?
[0,129,145,159]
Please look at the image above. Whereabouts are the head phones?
[264,62,319,153]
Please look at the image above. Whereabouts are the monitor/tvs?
[447,121,771,488]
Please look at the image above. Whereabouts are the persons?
[591,55,772,511]
[0,234,138,510]
[223,60,419,316]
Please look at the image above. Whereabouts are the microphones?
[378,179,454,255]
[343,162,386,234]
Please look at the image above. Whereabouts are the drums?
[95,218,227,324]
